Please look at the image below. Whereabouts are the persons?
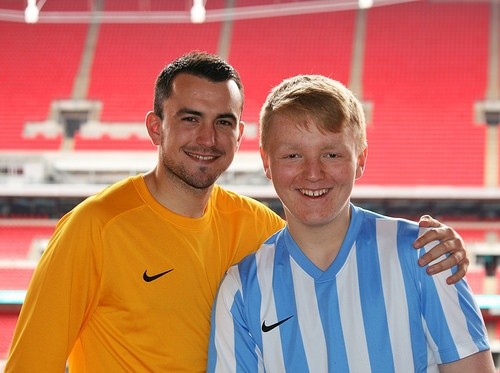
[205,74,497,373]
[4,49,470,373]
